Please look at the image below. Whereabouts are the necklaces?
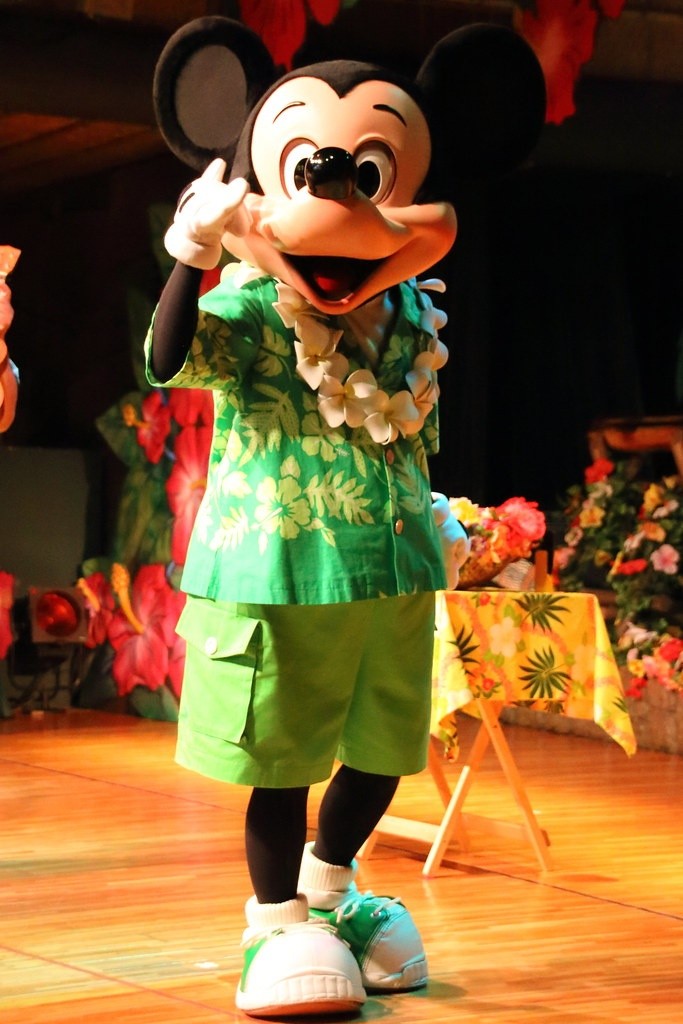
[273,280,447,446]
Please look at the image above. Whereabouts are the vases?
[457,563,508,587]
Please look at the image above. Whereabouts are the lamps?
[6,584,86,717]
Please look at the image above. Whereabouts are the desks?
[356,590,636,876]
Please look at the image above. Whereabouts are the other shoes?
[298,839,429,991]
[235,894,365,1016]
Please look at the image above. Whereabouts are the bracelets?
[0,353,9,376]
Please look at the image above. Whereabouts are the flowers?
[218,260,449,447]
[446,497,547,568]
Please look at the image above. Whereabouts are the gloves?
[164,157,249,269]
[431,491,470,590]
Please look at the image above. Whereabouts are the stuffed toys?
[140,17,547,1017]
